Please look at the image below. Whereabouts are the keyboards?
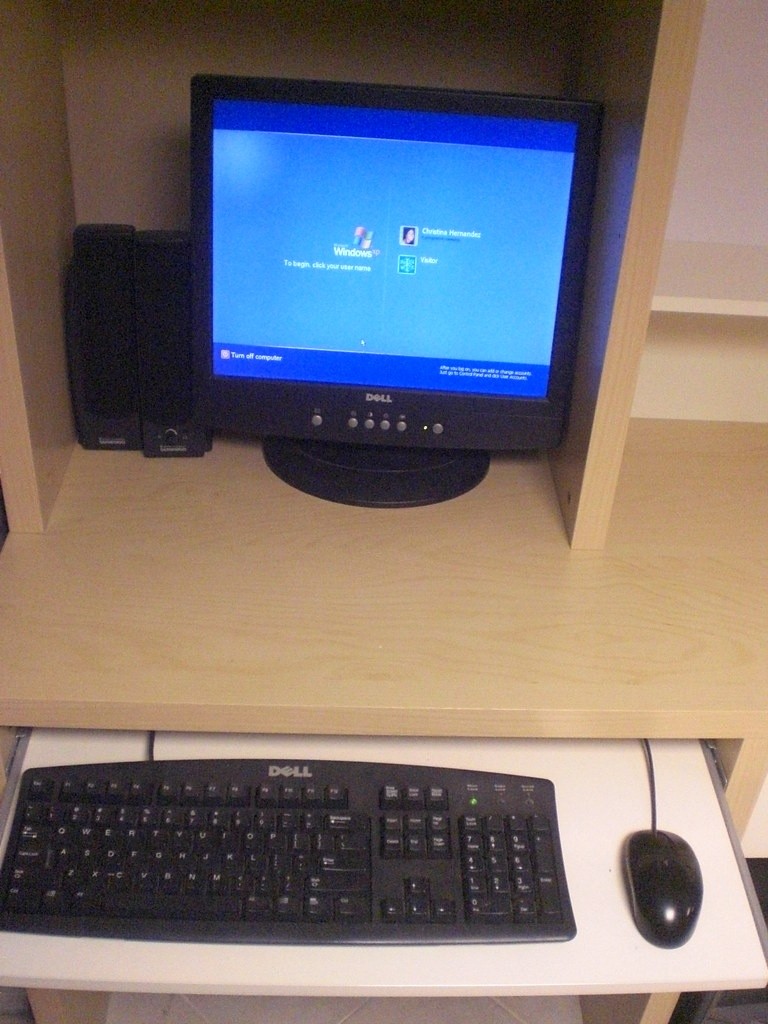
[0,759,577,946]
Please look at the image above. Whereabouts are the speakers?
[69,224,213,458]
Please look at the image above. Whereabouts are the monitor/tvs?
[188,72,606,509]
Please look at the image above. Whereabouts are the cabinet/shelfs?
[0,0,768,1024]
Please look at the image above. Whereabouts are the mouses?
[625,829,704,949]
